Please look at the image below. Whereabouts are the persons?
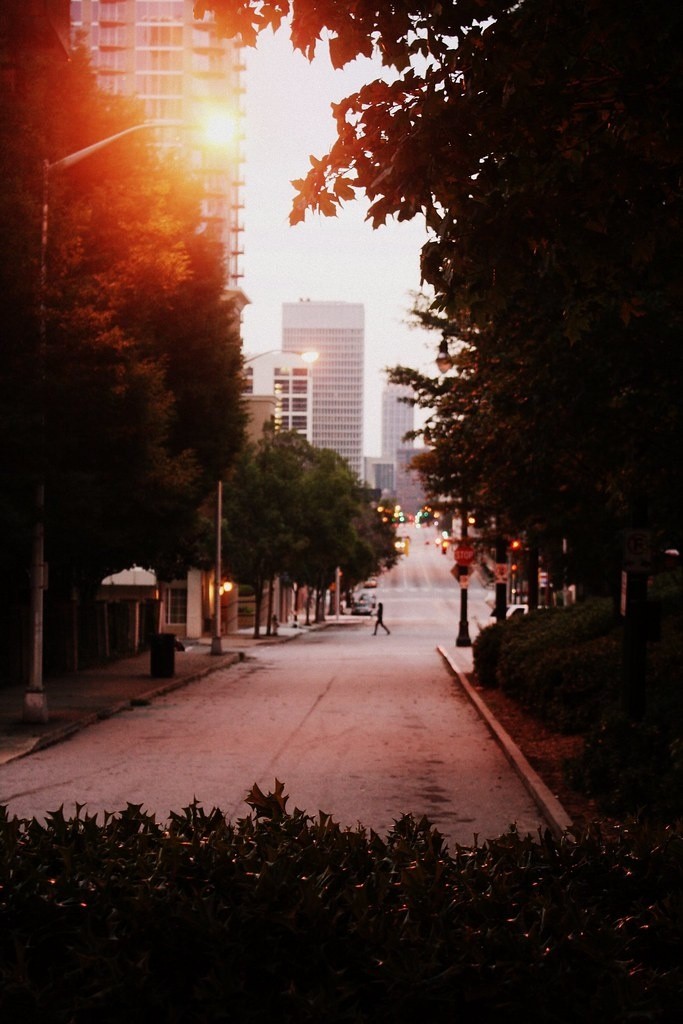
[372,602,391,635]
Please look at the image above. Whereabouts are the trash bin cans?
[149,633,175,679]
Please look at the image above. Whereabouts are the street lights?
[21,104,242,727]
[211,346,322,656]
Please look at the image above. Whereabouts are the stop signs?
[453,544,476,567]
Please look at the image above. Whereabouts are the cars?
[487,603,549,626]
[352,599,372,615]
[363,577,377,588]
[359,593,376,608]
